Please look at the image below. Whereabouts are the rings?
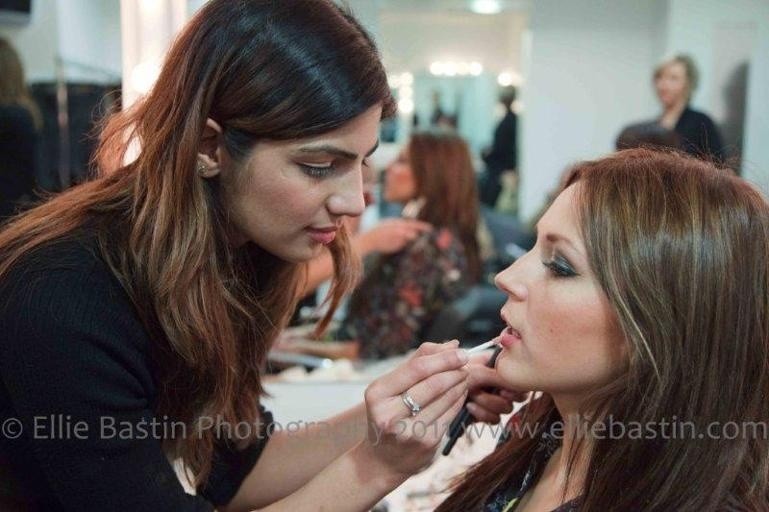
[399,390,420,419]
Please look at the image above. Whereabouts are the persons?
[641,52,725,169]
[0,0,528,511]
[285,214,431,298]
[0,35,48,227]
[427,143,769,511]
[279,131,490,361]
[478,83,515,219]
